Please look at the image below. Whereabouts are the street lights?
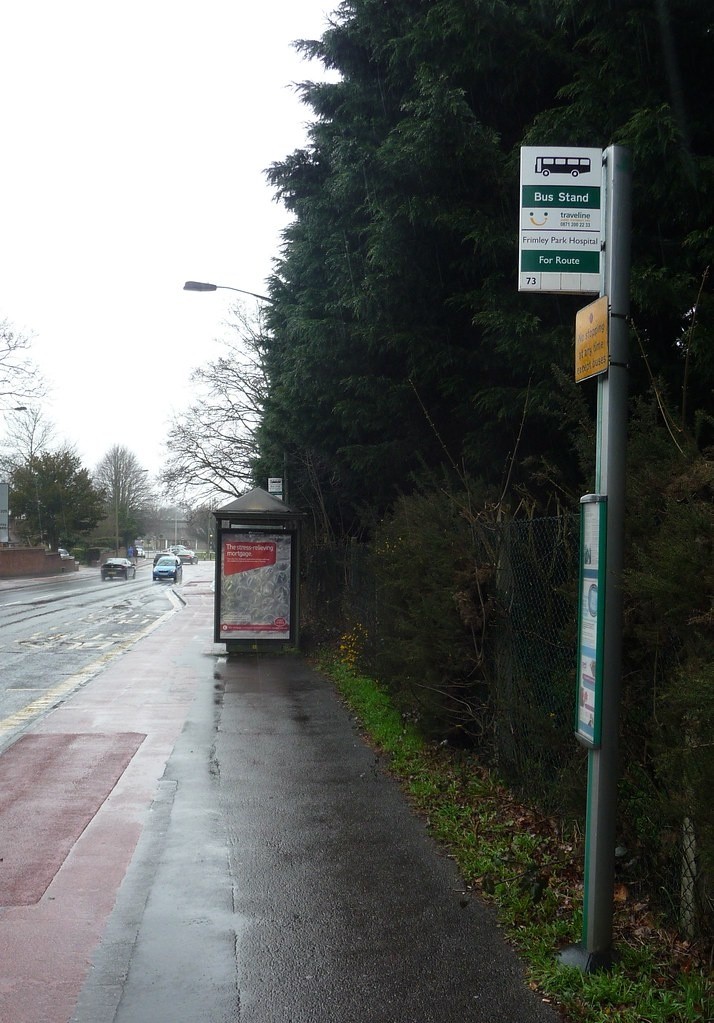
[115,469,149,557]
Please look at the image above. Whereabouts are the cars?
[58,548,69,558]
[166,544,199,565]
[152,555,183,582]
[153,551,176,569]
[100,557,137,581]
[136,547,145,558]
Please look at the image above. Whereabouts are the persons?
[128,545,138,564]
